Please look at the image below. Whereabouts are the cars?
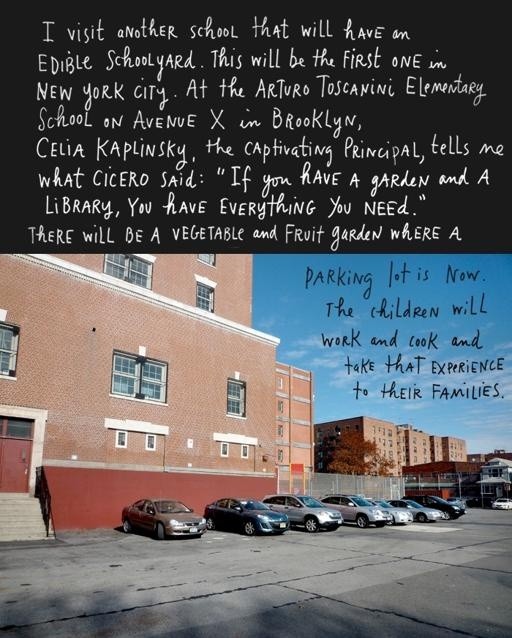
[122,498,206,540]
[204,494,466,536]
[490,498,511,510]
